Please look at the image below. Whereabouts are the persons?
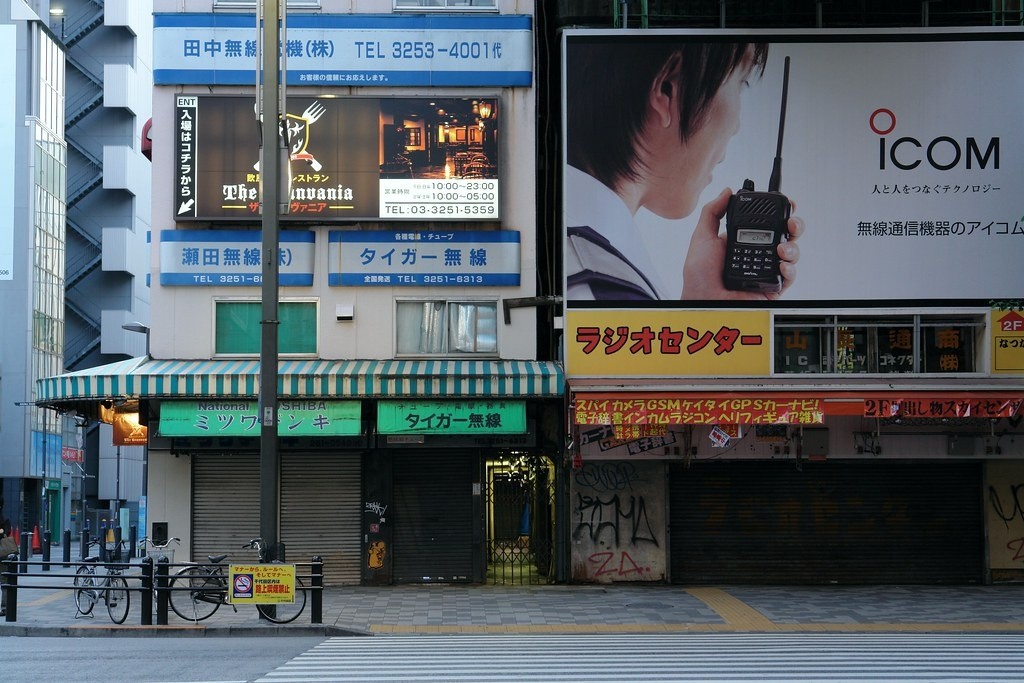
[567,36,803,301]
[1,510,11,616]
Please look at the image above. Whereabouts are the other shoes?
[0,609,6,616]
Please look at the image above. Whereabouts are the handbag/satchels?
[0,533,19,560]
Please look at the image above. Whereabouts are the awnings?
[567,378,1024,461]
[34,353,564,413]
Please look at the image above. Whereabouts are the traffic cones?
[32,525,40,550]
[9,525,13,538]
[14,525,23,548]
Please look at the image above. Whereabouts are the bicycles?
[138,536,181,613]
[167,537,307,623]
[73,538,131,624]
